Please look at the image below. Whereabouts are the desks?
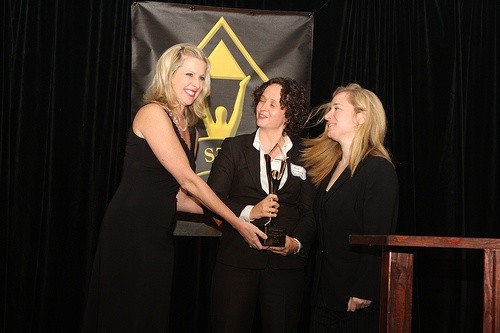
[348,234,500,333]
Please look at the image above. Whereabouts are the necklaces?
[173,116,187,131]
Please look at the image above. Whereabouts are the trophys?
[257,143,285,247]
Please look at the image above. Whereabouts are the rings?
[249,245,253,248]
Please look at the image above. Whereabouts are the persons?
[80,43,269,333]
[299,83,400,333]
[205,77,318,333]
